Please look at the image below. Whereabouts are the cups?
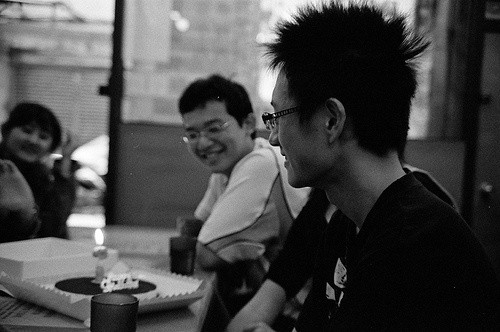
[90,293,140,332]
[168,236,198,276]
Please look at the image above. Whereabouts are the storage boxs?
[0,235,119,296]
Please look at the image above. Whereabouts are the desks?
[0,222,218,332]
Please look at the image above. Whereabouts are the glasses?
[262,96,328,131]
[182,112,247,147]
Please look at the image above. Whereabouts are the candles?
[93,228,108,284]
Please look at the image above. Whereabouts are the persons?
[0,102,81,243]
[178,72,313,332]
[222,0,500,332]
